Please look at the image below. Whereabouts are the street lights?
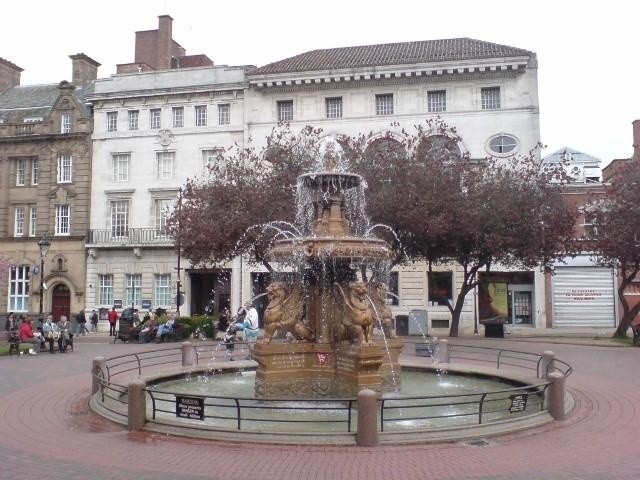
[174,184,191,316]
[37,232,53,349]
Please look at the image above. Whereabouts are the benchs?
[0,308,265,358]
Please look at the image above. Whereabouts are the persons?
[5,302,260,362]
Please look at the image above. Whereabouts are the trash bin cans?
[485,323,504,337]
[395,315,408,336]
[119,317,133,337]
